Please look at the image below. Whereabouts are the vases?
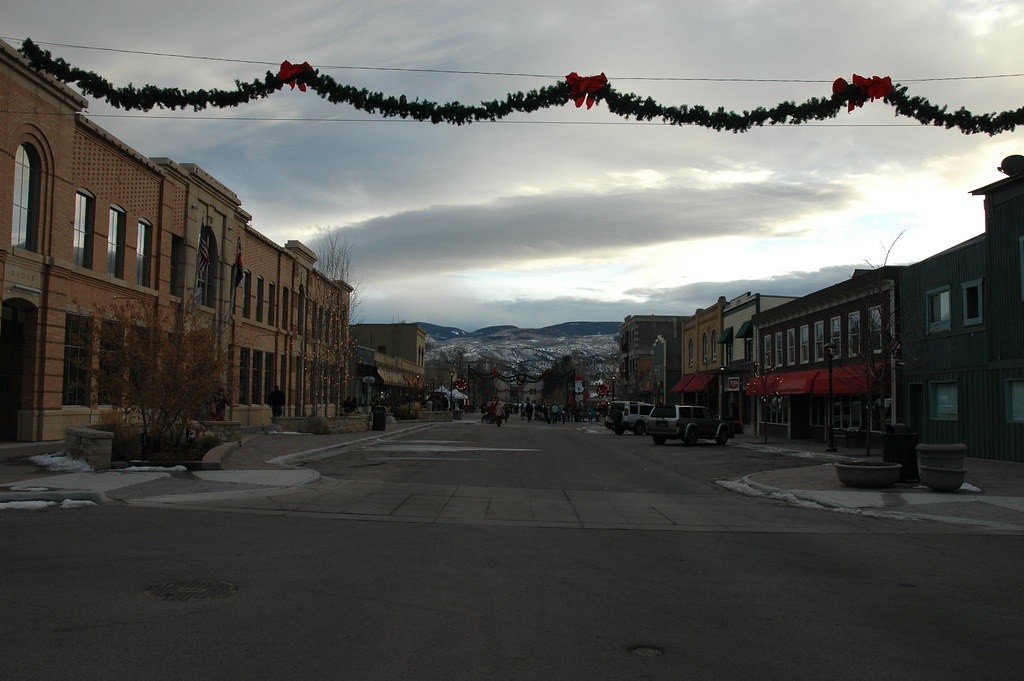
[921,467,966,493]
[915,443,967,481]
[834,458,907,488]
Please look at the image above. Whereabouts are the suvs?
[643,404,734,447]
[605,400,653,437]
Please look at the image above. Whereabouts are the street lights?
[415,373,420,402]
[717,365,730,427]
[653,362,662,405]
[822,340,840,454]
[611,374,616,401]
[448,370,454,410]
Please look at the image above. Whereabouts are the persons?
[214,388,229,421]
[370,394,449,413]
[268,386,285,416]
[488,399,609,427]
[344,396,358,415]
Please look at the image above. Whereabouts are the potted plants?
[881,422,919,483]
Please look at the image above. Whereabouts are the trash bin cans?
[882,423,920,485]
[372,406,386,431]
